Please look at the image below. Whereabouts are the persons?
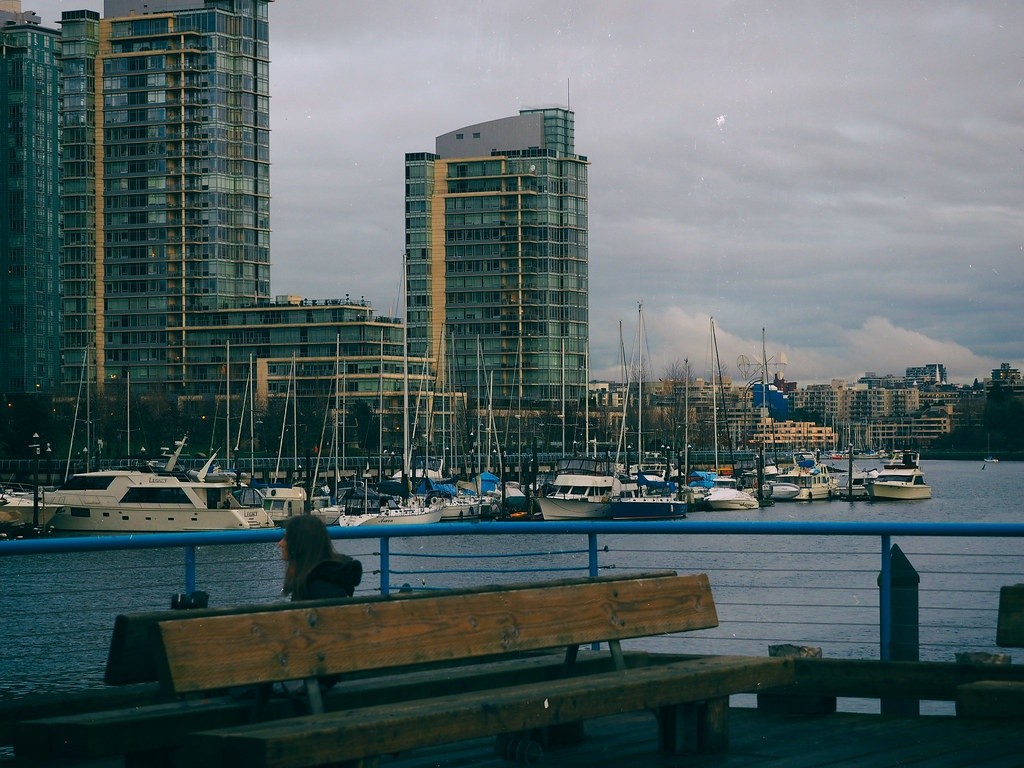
[228,513,363,699]
[225,490,234,509]
[800,454,805,462]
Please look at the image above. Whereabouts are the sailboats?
[0,301,834,554]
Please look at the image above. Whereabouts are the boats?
[984,458,999,463]
[872,449,932,500]
[836,468,879,501]
